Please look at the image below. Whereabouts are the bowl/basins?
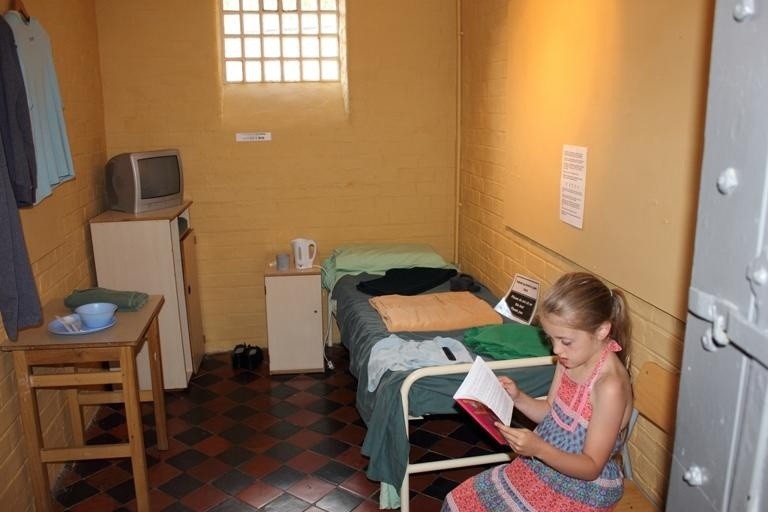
[76,301,118,327]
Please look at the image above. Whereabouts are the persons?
[439,272,633,512]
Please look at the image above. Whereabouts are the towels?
[64,288,148,311]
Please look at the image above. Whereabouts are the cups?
[277,254,290,271]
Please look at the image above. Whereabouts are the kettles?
[290,239,317,269]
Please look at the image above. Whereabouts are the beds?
[328,255,558,512]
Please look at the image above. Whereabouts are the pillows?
[332,244,449,271]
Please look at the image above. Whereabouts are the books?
[453,355,514,447]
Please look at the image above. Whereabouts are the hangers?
[9,0,30,21]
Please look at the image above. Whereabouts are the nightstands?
[264,258,325,375]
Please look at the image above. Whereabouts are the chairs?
[613,362,679,512]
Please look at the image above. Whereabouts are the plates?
[47,313,118,335]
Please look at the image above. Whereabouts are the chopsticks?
[54,314,78,333]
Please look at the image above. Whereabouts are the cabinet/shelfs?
[88,200,206,390]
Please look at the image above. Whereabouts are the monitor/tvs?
[102,147,184,214]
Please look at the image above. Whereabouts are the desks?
[0,295,169,512]
